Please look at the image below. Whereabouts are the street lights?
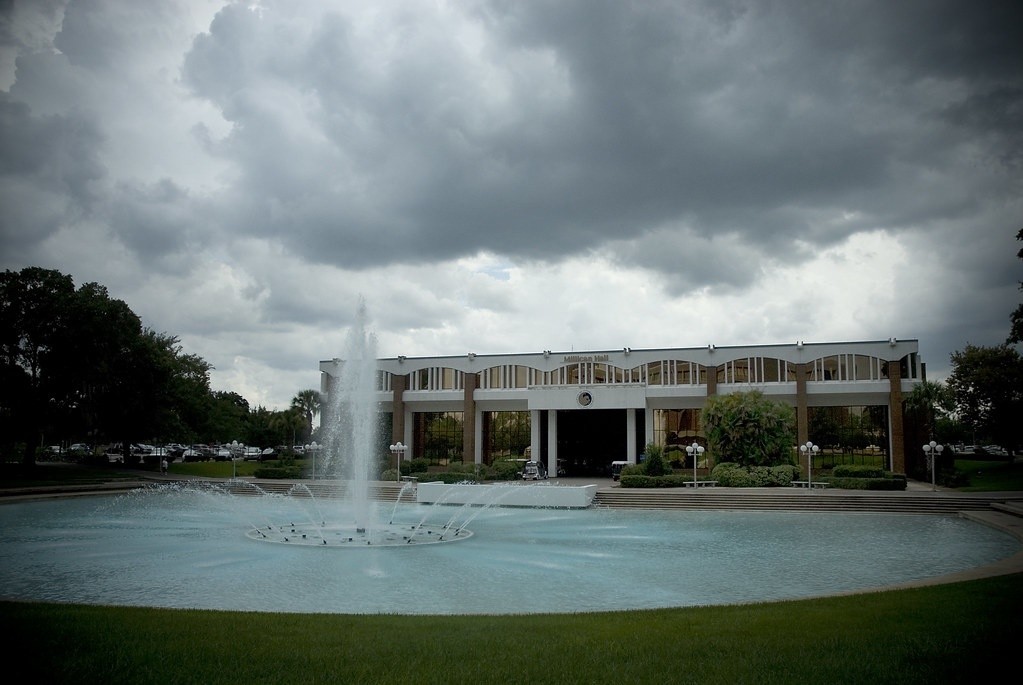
[922,441,943,491]
[390,442,408,483]
[800,442,819,488]
[305,441,323,480]
[226,440,244,478]
[686,443,704,487]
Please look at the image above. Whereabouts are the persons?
[161,457,168,476]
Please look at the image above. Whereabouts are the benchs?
[683,481,718,488]
[790,481,830,489]
[401,476,418,483]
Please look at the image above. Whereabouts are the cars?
[950,444,1009,453]
[46,442,303,462]
[522,461,547,481]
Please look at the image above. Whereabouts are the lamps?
[796,341,804,350]
[398,356,406,363]
[543,350,551,358]
[623,348,631,355]
[333,358,341,365]
[888,337,897,347]
[468,353,476,361]
[708,344,715,353]
